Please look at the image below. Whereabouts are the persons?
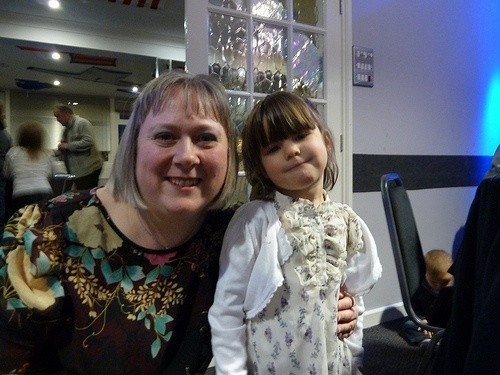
[5,123,57,207]
[434,177,500,375]
[0,68,360,375]
[401,251,454,351]
[208,90,381,375]
[54,105,103,190]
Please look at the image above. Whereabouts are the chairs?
[380,173,454,375]
